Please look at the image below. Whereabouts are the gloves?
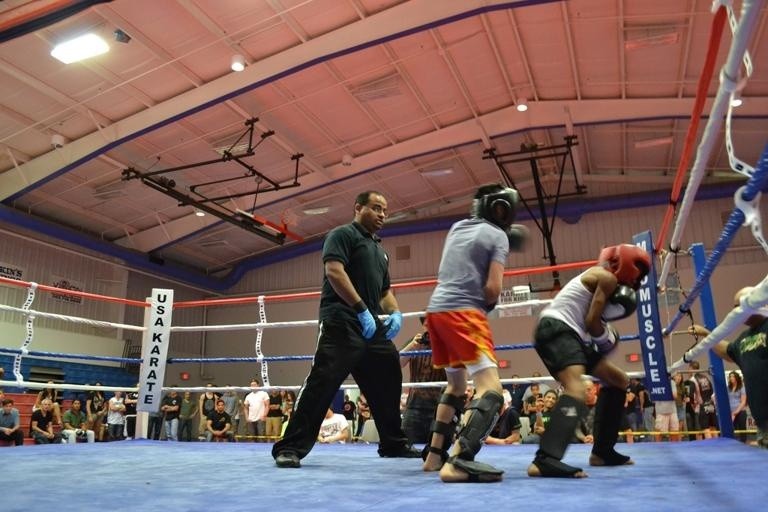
[601,285,639,320]
[593,322,621,353]
[504,224,530,253]
[381,311,405,339]
[355,311,378,341]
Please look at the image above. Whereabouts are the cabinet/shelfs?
[119,117,302,243]
[480,134,588,294]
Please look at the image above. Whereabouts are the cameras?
[416,331,430,345]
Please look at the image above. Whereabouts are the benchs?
[233,435,281,443]
[0,392,75,446]
[0,354,136,407]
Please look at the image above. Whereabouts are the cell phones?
[537,393,542,398]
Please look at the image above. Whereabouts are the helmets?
[471,182,522,230]
[597,239,653,286]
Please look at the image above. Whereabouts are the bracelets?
[353,299,368,314]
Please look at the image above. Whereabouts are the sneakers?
[377,439,421,459]
[274,450,300,468]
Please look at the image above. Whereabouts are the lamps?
[49,33,112,65]
[731,97,745,109]
[232,54,245,72]
[303,206,329,215]
[196,208,206,218]
[515,97,529,112]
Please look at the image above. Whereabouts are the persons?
[688,286,768,447]
[452,385,474,443]
[486,372,598,444]
[528,243,652,478]
[317,408,349,444]
[424,184,529,482]
[0,367,296,447]
[356,390,371,438]
[655,368,679,442]
[342,395,357,436]
[623,378,638,432]
[727,371,747,442]
[675,361,717,439]
[633,378,654,431]
[271,189,425,467]
